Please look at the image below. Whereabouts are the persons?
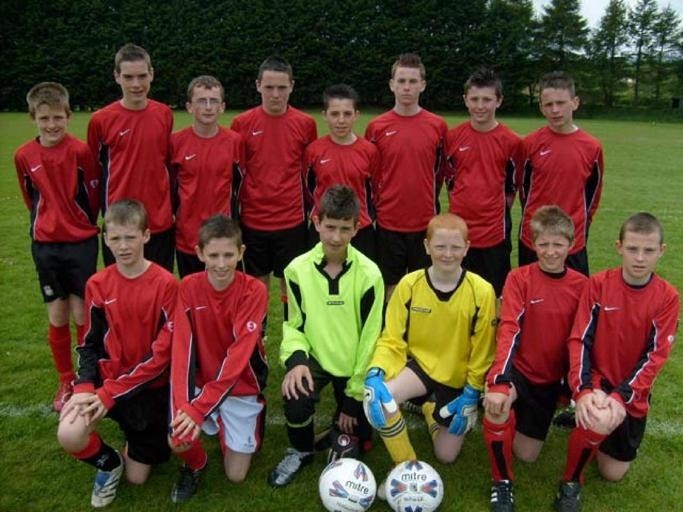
[446,69,522,302]
[169,215,269,507]
[14,82,99,411]
[302,85,382,264]
[524,74,604,275]
[58,199,177,507]
[553,212,679,511]
[482,204,589,511]
[363,212,497,501]
[364,53,448,299]
[230,57,319,360]
[271,184,385,488]
[86,42,175,274]
[170,76,243,276]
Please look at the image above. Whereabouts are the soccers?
[385,459,444,512]
[318,457,378,512]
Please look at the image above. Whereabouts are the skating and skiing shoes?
[264,446,317,489]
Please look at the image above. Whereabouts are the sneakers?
[489,476,517,512]
[166,462,212,505]
[49,380,70,413]
[377,470,393,502]
[87,443,127,511]
[551,477,582,512]
